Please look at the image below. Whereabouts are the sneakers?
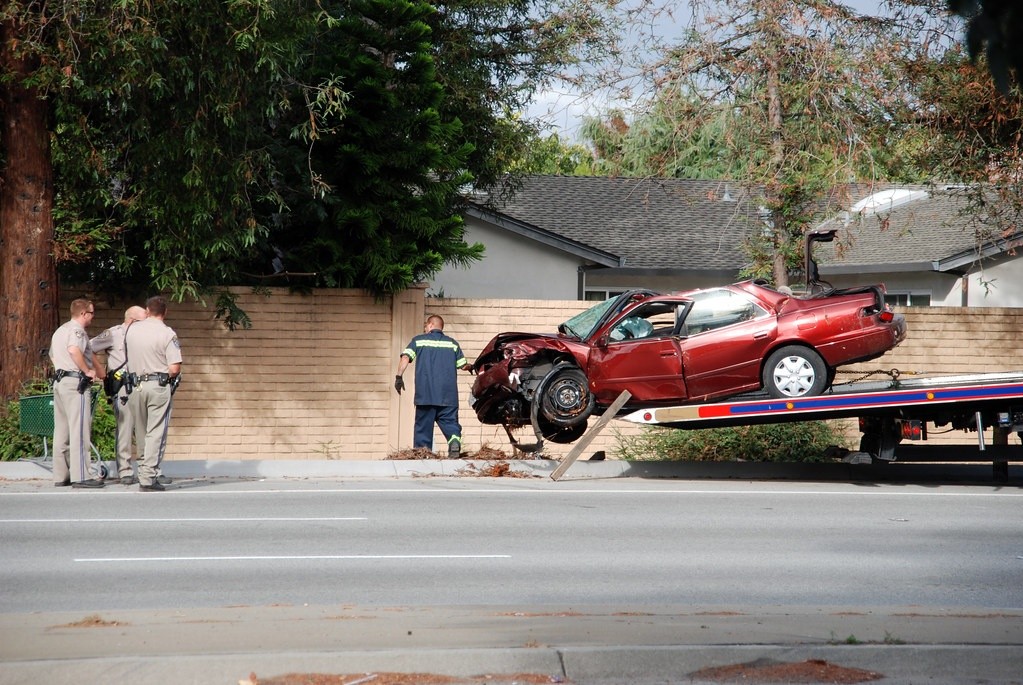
[156,475,172,484]
[120,475,134,484]
[139,480,165,492]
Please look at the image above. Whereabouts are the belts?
[138,375,171,383]
[53,371,82,378]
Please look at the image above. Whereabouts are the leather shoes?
[72,479,106,488]
[448,438,461,459]
[55,479,70,486]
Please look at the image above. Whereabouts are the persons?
[395,317,476,459]
[89,296,182,491]
[49,296,105,489]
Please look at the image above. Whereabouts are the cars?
[472,228,906,444]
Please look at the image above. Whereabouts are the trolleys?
[18,383,107,479]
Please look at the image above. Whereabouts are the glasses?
[81,311,94,315]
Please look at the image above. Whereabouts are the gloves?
[395,375,405,395]
